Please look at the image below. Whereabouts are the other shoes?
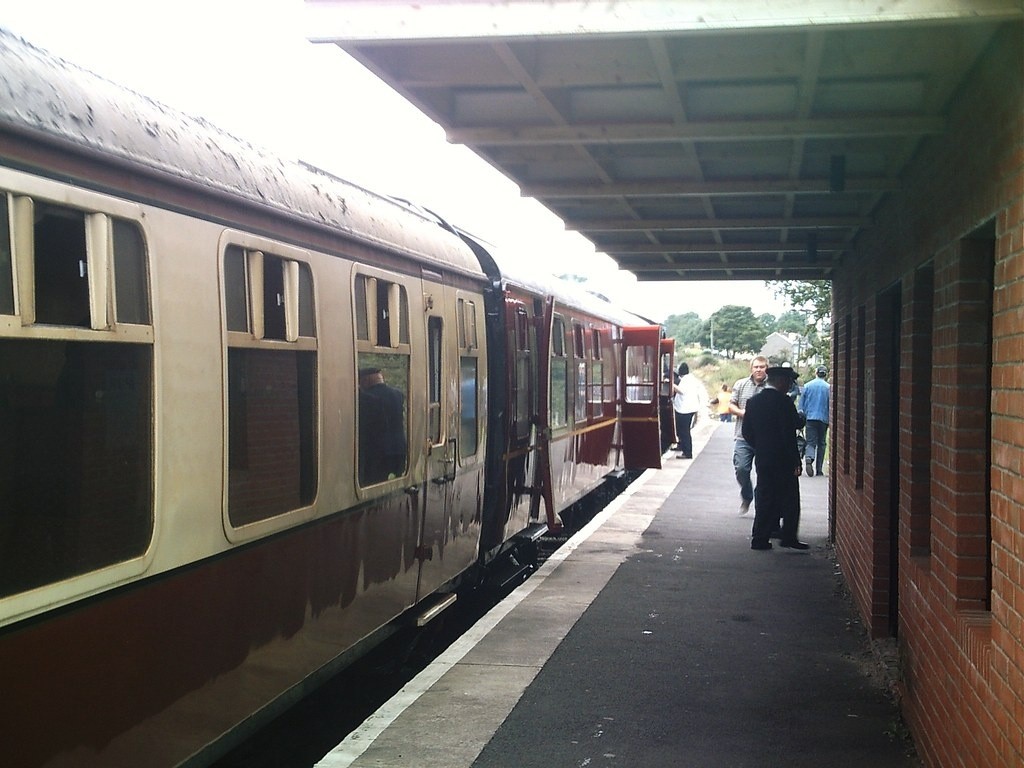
[805,456,813,477]
[738,498,753,515]
[670,447,683,451]
[676,454,692,458]
[780,540,809,549]
[751,542,772,549]
[816,472,823,476]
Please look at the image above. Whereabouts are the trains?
[0,29,678,766]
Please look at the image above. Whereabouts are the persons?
[461,368,477,447]
[727,355,773,516]
[739,366,811,549]
[797,366,830,476]
[356,368,411,489]
[662,361,680,395]
[709,385,734,422]
[664,361,700,460]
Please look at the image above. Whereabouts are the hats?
[818,365,827,373]
[678,362,688,375]
[764,366,800,385]
